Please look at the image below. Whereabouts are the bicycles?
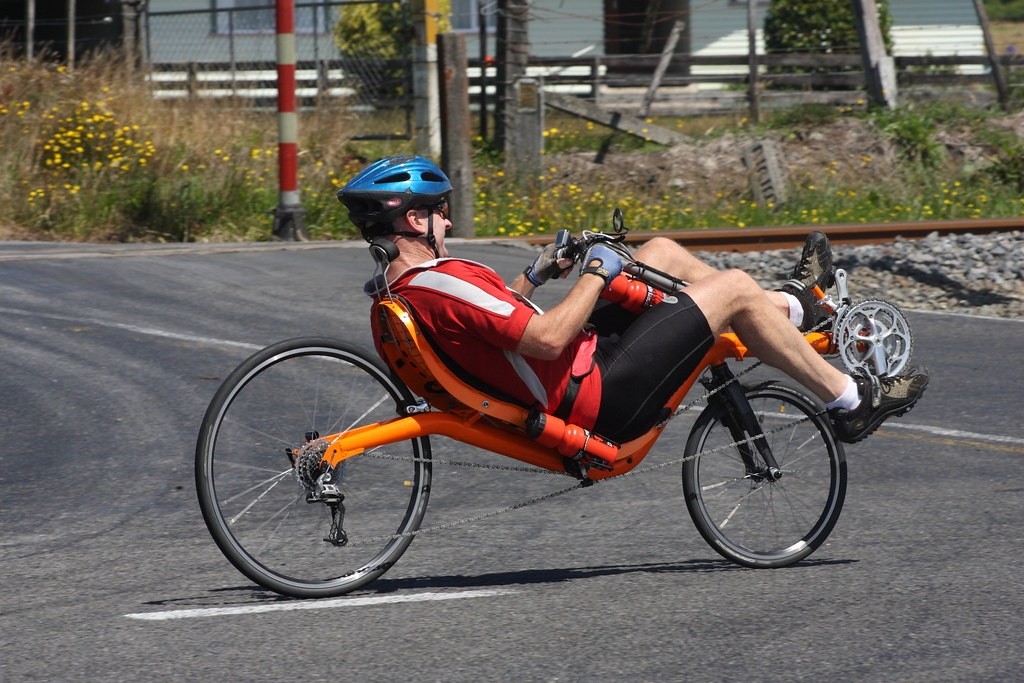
[192,210,914,597]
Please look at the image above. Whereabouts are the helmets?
[337,155,454,259]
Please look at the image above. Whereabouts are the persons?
[335,154,930,445]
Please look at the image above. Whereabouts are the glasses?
[434,197,450,218]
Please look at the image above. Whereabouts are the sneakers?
[774,231,833,331]
[825,365,931,444]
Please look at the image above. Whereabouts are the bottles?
[598,272,671,314]
[526,411,618,469]
